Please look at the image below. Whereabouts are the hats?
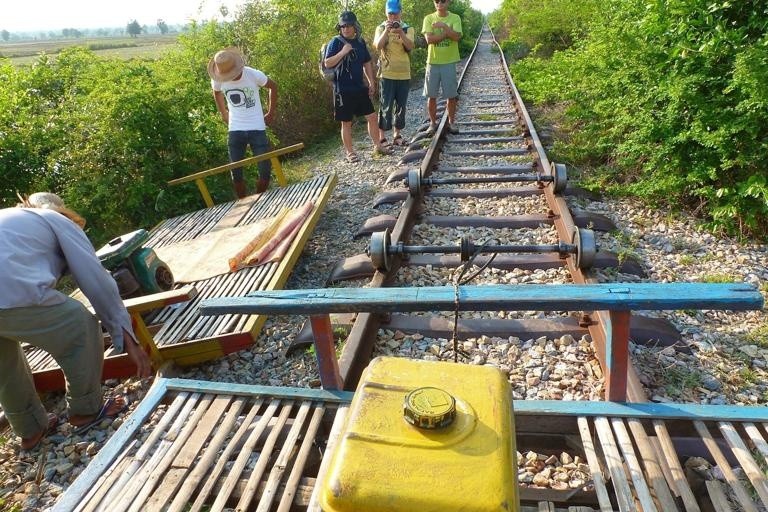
[338,12,357,28]
[208,47,244,83]
[386,0,401,14]
[16,191,85,230]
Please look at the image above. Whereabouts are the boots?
[255,180,269,192]
[234,180,246,199]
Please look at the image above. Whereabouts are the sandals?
[345,152,358,162]
[374,134,409,154]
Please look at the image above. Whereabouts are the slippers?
[33,412,59,451]
[71,395,129,435]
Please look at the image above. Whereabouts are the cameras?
[393,21,400,28]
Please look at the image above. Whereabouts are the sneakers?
[447,123,459,133]
[427,121,439,132]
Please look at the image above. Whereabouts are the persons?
[0,192,153,453]
[207,50,277,198]
[420,0,464,134]
[324,12,395,162]
[373,0,415,151]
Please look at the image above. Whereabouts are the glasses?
[435,0,446,3]
[340,23,353,28]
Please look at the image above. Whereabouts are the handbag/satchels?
[318,36,352,81]
